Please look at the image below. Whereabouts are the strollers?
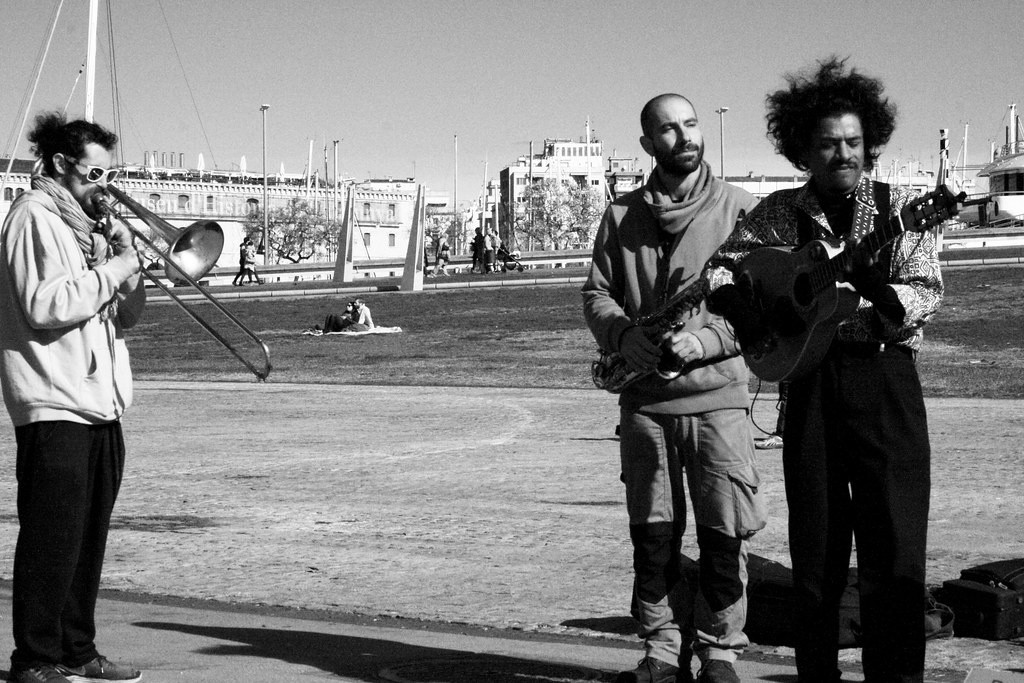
[494,242,524,273]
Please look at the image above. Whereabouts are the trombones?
[90,184,271,379]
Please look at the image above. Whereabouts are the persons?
[232,238,259,286]
[581,94,760,683]
[469,227,502,275]
[323,297,373,332]
[0,116,148,683]
[698,56,943,683]
[431,234,450,276]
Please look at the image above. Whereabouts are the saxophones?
[591,208,746,394]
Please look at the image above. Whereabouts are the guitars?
[732,184,963,384]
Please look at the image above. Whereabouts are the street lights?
[259,102,271,265]
[713,107,730,182]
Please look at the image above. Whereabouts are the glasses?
[347,304,353,307]
[64,155,119,185]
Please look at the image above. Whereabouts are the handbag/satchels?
[931,558,1024,641]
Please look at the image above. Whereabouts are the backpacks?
[836,566,956,649]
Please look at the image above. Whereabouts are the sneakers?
[55,653,143,683]
[609,655,694,683]
[695,658,741,683]
[6,661,73,683]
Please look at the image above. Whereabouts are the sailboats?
[872,102,1024,231]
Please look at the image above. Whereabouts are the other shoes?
[239,284,245,286]
[233,281,237,286]
